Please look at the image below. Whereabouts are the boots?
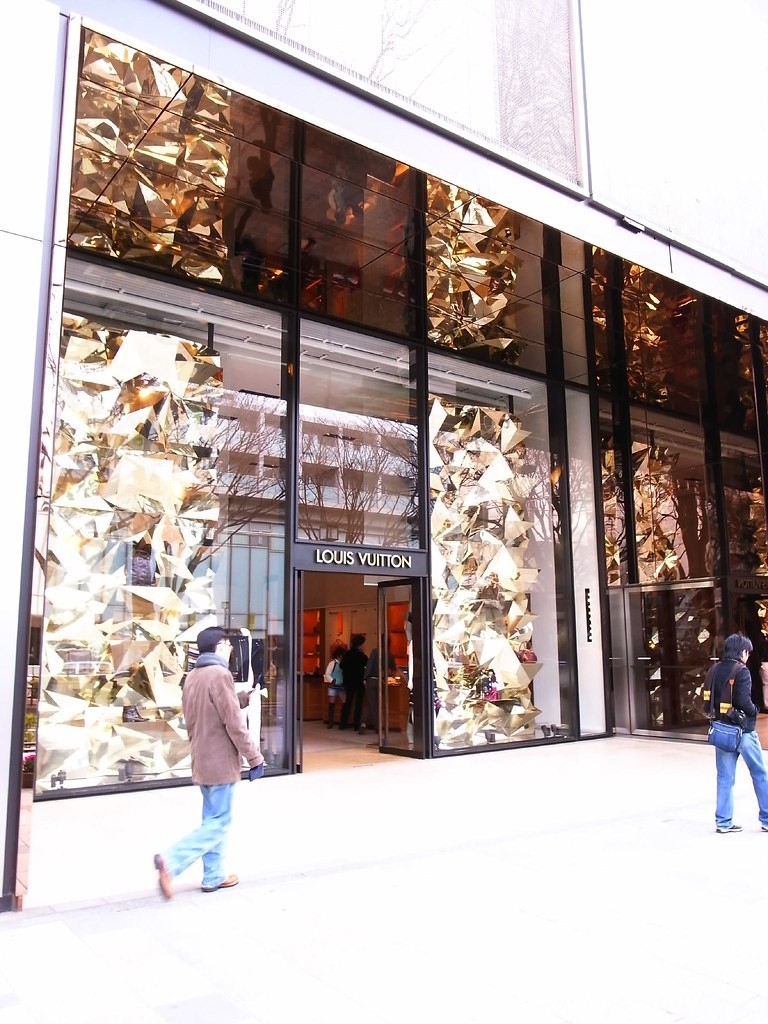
[326,703,335,729]
[339,703,345,729]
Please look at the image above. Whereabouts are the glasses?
[217,642,233,651]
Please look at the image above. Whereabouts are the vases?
[22,771,35,789]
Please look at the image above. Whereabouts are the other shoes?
[201,874,238,892]
[354,726,360,731]
[375,725,379,733]
[154,853,173,898]
[359,726,365,734]
[339,725,349,729]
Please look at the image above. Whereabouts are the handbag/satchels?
[324,675,332,682]
[708,721,742,752]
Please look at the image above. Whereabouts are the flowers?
[23,754,36,773]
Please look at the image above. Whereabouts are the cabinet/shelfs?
[297,600,414,734]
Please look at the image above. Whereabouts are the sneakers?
[716,824,743,833]
[761,822,768,831]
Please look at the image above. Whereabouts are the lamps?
[50,771,65,787]
[124,762,133,779]
[434,735,440,751]
[541,724,550,737]
[485,731,490,744]
[261,749,276,767]
[550,724,557,736]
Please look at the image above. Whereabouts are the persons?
[701,632,768,833]
[323,646,347,730]
[339,633,369,730]
[358,632,408,735]
[153,626,268,901]
